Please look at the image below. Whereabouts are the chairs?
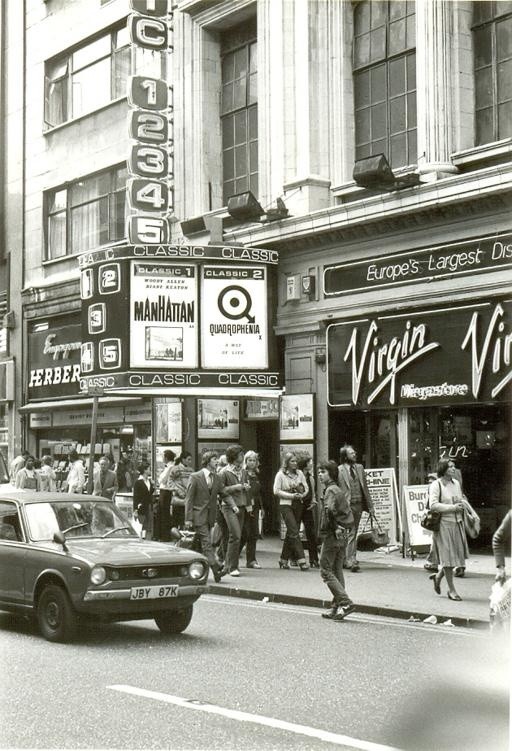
[1,516,22,542]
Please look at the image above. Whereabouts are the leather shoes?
[423,564,464,602]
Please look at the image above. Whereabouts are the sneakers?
[321,603,355,620]
[214,562,262,583]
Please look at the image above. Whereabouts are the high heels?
[278,560,319,571]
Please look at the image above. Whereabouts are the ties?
[350,467,355,480]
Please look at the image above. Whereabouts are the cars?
[1,492,210,643]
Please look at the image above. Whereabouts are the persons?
[428,457,470,601]
[336,446,375,571]
[491,507,512,587]
[10,444,263,583]
[290,455,320,569]
[273,453,309,571]
[424,468,467,578]
[315,461,356,622]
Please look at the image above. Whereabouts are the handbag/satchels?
[419,512,440,533]
[171,495,185,507]
[174,526,196,551]
[356,528,390,552]
